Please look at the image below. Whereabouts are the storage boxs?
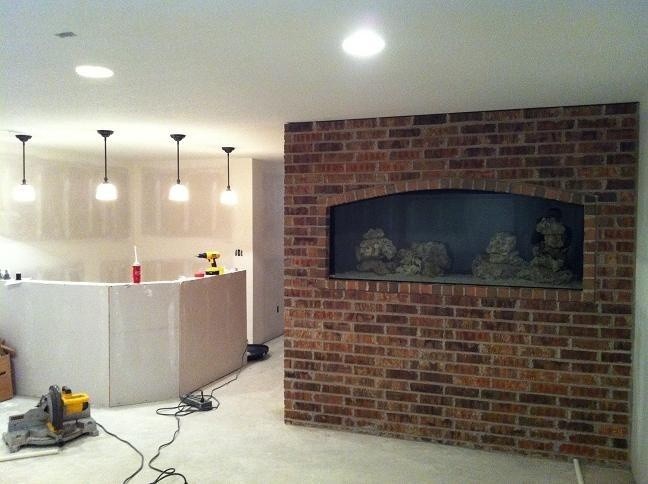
[0,341,19,401]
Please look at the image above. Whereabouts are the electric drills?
[194,252,224,275]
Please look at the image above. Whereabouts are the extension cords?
[180,393,213,410]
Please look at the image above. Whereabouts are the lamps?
[219,146,239,207]
[95,129,119,200]
[167,133,192,202]
[11,133,37,203]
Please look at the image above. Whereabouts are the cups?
[132,263,142,284]
